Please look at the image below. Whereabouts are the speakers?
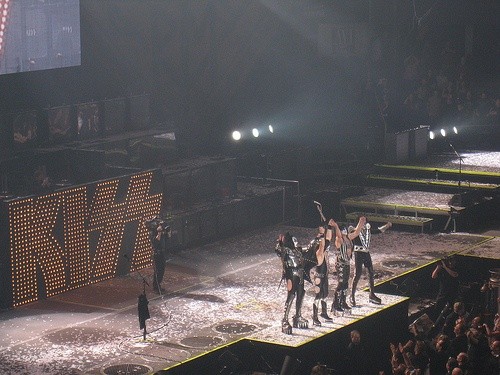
[449,191,472,207]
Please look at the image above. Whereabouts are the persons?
[149,226,171,296]
[388,51,500,125]
[275,215,500,375]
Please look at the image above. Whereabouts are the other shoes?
[369,294,381,305]
[281,319,292,335]
[292,315,308,329]
[349,295,356,307]
[331,298,344,317]
[153,287,166,294]
[340,298,352,316]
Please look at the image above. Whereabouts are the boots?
[311,303,321,326]
[320,300,333,324]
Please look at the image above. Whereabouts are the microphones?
[314,200,321,205]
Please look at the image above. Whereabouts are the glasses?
[152,224,158,226]
